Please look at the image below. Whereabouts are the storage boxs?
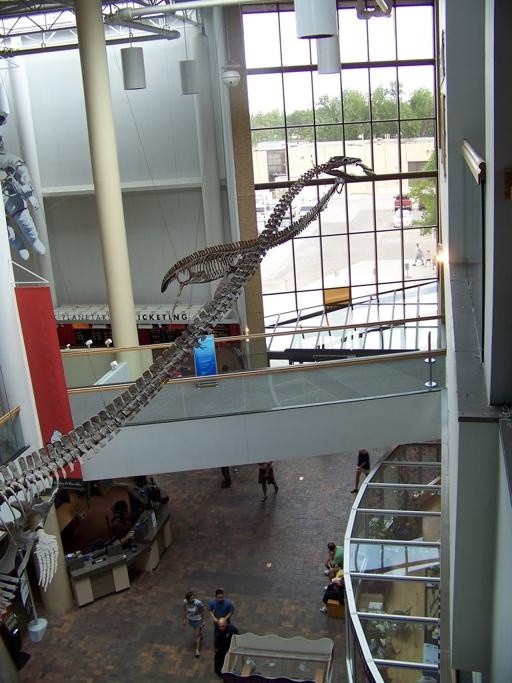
[325,597,346,621]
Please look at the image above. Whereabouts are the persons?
[220,464,232,489]
[256,458,278,500]
[212,615,239,677]
[351,447,372,494]
[0,134,48,261]
[207,588,235,631]
[412,242,425,265]
[325,541,346,576]
[424,249,432,263]
[183,590,208,657]
[321,576,343,615]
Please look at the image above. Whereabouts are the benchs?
[18,571,34,617]
[194,333,219,377]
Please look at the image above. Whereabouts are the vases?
[360,603,418,682]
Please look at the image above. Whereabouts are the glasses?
[67,337,114,349]
[118,0,342,94]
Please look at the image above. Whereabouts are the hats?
[393,193,425,228]
[256,196,327,227]
